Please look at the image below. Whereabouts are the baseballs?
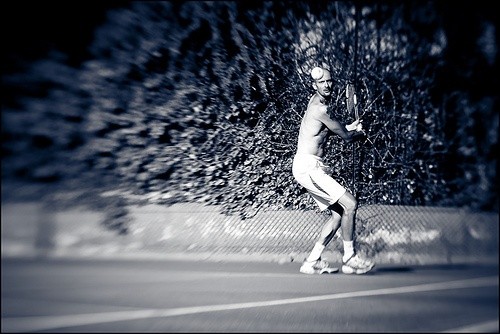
[311,67,323,79]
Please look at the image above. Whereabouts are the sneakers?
[341,252,375,275]
[300,257,339,274]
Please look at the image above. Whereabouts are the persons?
[292,66,376,274]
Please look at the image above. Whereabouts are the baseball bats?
[353,94,359,120]
